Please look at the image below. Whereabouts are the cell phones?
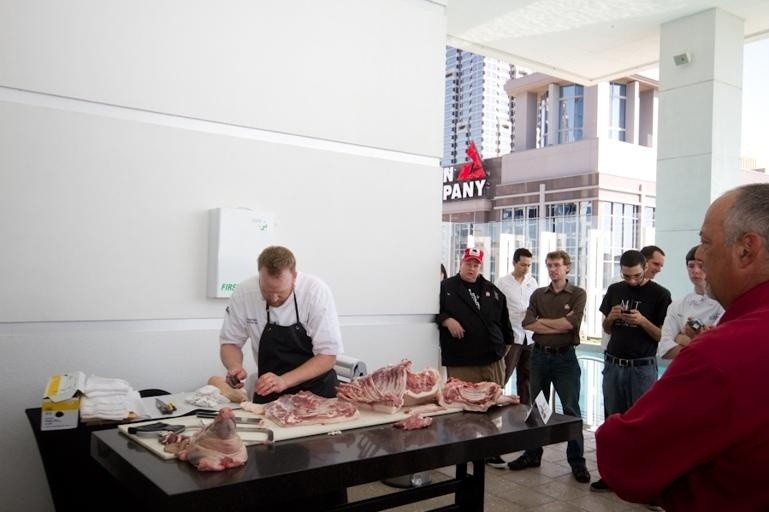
[621,309,632,314]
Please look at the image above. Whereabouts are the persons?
[507,251,591,484]
[602,244,666,361]
[219,246,349,512]
[593,182,769,510]
[440,263,448,281]
[656,244,726,364]
[492,247,540,408]
[588,249,674,510]
[679,315,718,341]
[440,246,515,470]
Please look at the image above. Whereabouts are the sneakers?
[572,465,590,483]
[590,479,609,492]
[487,455,507,469]
[508,455,541,470]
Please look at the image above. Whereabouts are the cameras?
[687,317,703,332]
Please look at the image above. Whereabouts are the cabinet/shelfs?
[26,390,171,512]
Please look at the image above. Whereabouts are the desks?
[92,385,583,511]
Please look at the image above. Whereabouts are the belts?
[606,356,653,367]
[534,343,570,354]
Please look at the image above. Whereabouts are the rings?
[270,380,275,386]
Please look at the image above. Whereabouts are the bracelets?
[534,314,540,320]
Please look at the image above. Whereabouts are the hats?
[463,248,484,263]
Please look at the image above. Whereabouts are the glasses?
[620,272,642,280]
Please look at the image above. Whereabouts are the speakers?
[673,51,691,66]
